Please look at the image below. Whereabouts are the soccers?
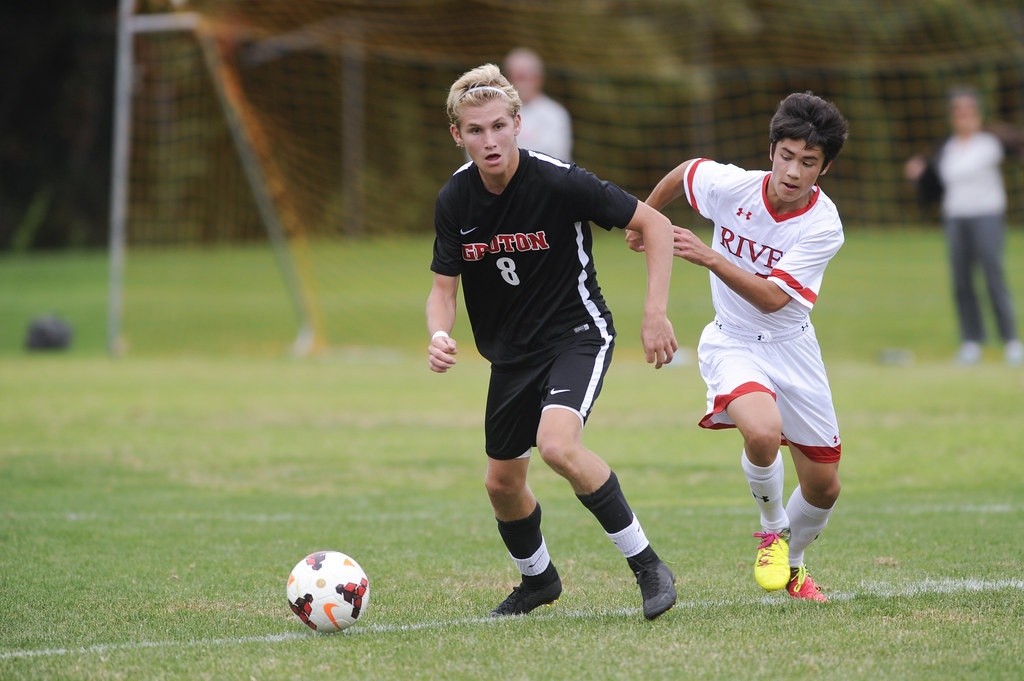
[286,550,370,634]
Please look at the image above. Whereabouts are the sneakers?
[754,530,827,604]
[490,567,562,617]
[636,557,678,618]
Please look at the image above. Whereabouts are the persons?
[900,82,1022,368]
[624,92,845,602]
[465,46,572,163]
[426,63,679,619]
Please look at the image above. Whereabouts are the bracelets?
[432,330,448,337]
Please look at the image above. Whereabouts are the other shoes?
[959,342,983,368]
[1005,339,1024,368]
